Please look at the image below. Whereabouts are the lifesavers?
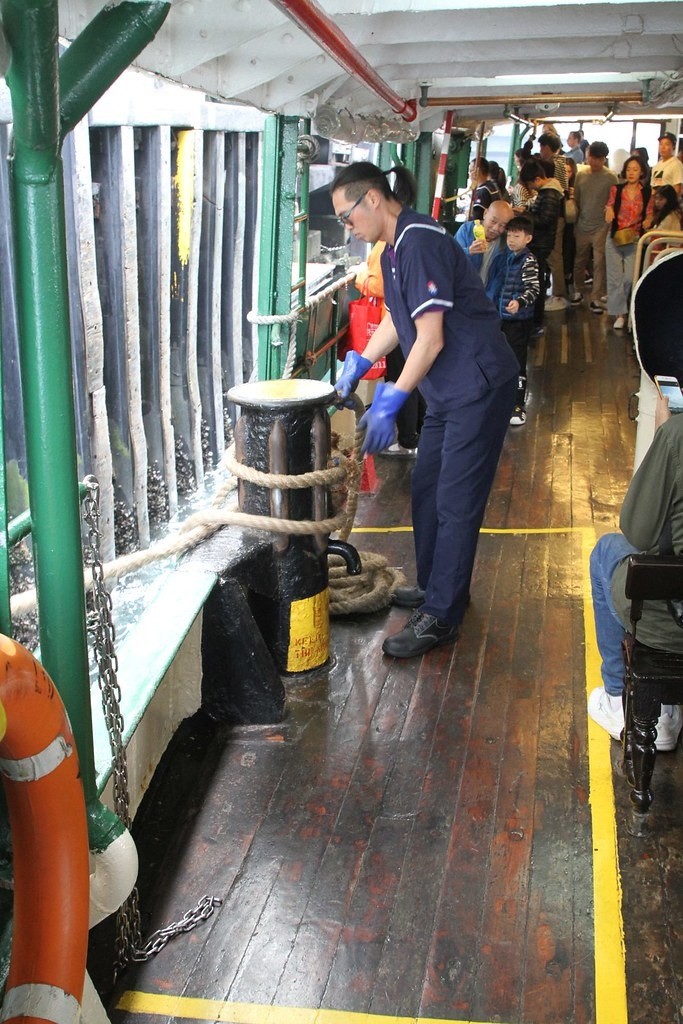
[1,631,94,1024]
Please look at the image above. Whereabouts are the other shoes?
[613,318,626,328]
[571,294,583,306]
[536,328,543,337]
[544,296,568,311]
[589,301,603,313]
[600,294,609,303]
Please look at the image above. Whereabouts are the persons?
[467,119,683,329]
[332,162,522,658]
[345,166,427,460]
[454,200,541,425]
[587,395,683,749]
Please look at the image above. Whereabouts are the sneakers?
[381,610,460,659]
[588,687,626,740]
[510,406,527,424]
[391,586,472,609]
[654,706,683,751]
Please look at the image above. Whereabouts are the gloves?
[333,350,373,410]
[356,382,411,455]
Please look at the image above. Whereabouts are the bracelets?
[530,134,536,140]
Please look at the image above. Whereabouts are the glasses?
[337,189,371,228]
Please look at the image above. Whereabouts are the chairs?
[617,556,683,837]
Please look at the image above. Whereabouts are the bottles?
[474,219,487,252]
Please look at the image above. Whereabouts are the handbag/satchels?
[348,275,387,381]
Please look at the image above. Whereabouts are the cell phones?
[654,375,683,413]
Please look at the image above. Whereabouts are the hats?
[658,132,677,142]
